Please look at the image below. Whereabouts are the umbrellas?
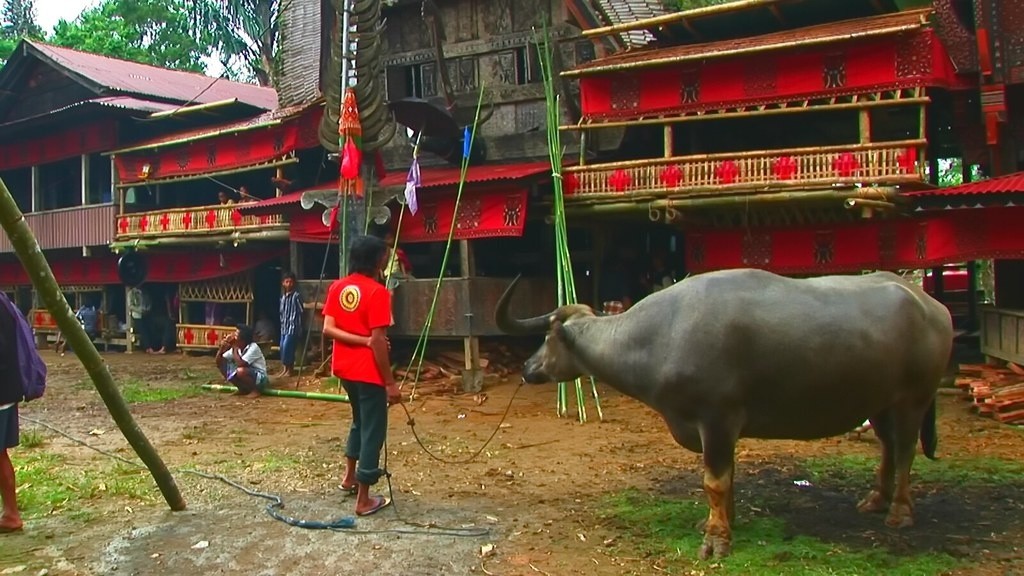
[385,96,464,165]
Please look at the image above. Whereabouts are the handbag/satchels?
[0,290,47,402]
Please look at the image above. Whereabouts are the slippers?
[359,495,392,516]
[0,527,16,533]
[337,484,359,491]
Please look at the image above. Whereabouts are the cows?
[495,269,953,559]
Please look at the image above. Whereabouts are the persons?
[217,190,235,205]
[214,323,268,399]
[278,273,306,378]
[238,185,256,203]
[135,282,179,355]
[321,235,403,514]
[53,301,99,355]
[0,292,35,533]
[377,226,415,281]
